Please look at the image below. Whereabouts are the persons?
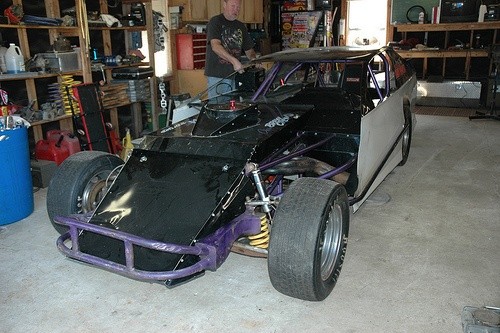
[204,0,258,107]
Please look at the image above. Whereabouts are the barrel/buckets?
[0,125,34,226]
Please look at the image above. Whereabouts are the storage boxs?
[112,67,154,79]
[38,51,79,71]
[28,160,56,187]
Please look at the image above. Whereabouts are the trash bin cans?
[0,125,34,226]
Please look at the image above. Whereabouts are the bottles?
[0,43,24,74]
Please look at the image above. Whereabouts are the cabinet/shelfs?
[395,21,500,81]
[173,0,263,25]
[0,0,160,145]
[173,32,208,70]
[175,62,273,100]
